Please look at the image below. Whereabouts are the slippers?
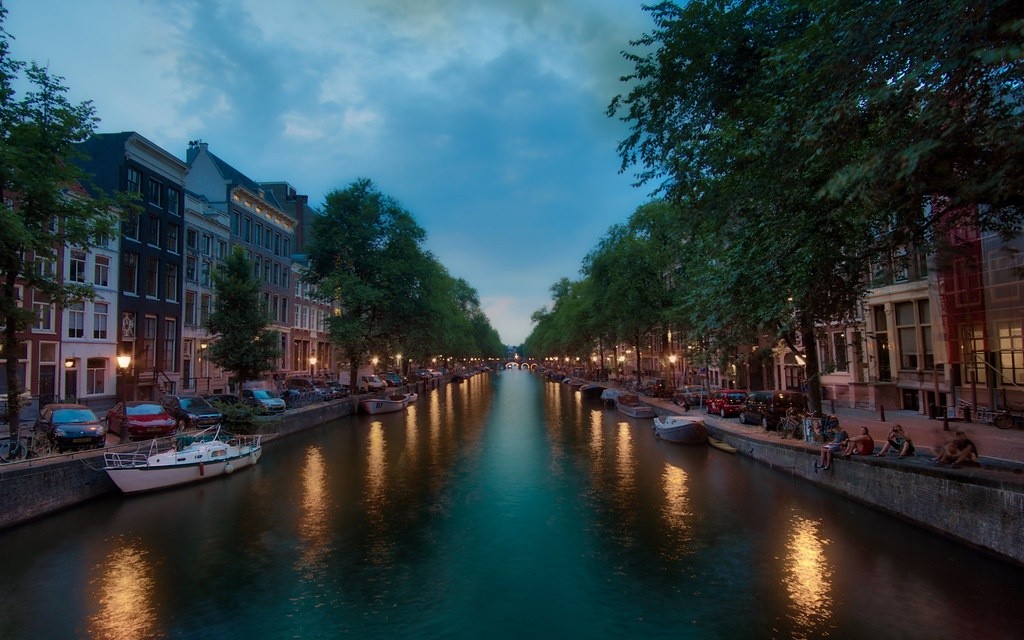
[874,453,885,457]
[898,454,904,459]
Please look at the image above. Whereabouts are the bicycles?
[776,404,841,442]
[0,423,52,463]
[995,402,1024,431]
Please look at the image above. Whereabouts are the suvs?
[737,390,808,431]
[705,388,749,418]
[157,393,228,432]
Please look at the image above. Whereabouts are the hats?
[955,430,964,436]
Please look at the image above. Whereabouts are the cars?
[105,400,179,442]
[279,362,491,403]
[239,387,287,416]
[644,377,667,398]
[202,393,247,409]
[33,403,107,453]
[674,384,710,407]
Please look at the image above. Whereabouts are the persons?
[874,424,915,459]
[930,431,979,469]
[813,424,874,472]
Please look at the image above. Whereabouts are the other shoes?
[816,465,825,469]
[935,460,946,465]
[949,463,961,469]
[821,466,830,471]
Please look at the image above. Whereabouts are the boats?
[405,390,418,403]
[653,414,708,446]
[533,360,657,420]
[707,435,739,455]
[102,422,264,498]
[359,393,411,416]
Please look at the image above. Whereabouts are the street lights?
[669,353,677,386]
[793,350,807,393]
[309,356,317,385]
[117,352,132,444]
